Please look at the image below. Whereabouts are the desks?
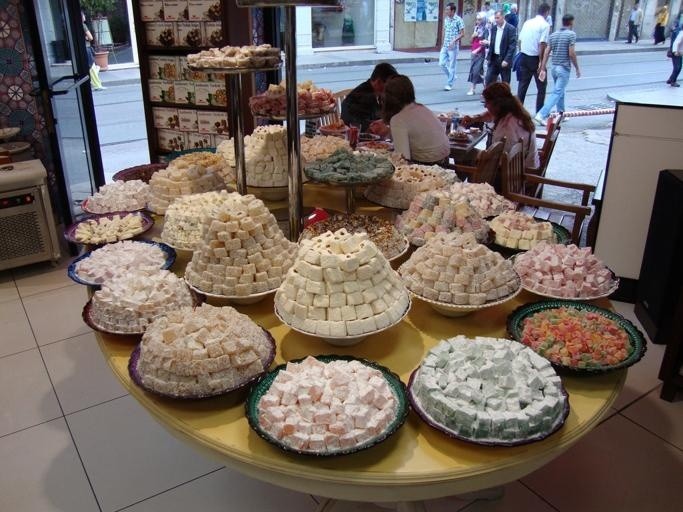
[82,161,629,512]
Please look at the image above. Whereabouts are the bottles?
[449,107,461,133]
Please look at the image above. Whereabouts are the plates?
[357,141,394,152]
[0,141,31,153]
[447,135,471,142]
[0,126,19,138]
[356,132,380,141]
[63,147,650,457]
[320,124,349,132]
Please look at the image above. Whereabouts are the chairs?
[522,121,560,203]
[500,138,597,246]
[532,110,563,153]
[443,138,505,185]
[317,102,338,128]
[330,88,352,121]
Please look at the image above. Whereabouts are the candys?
[76,240,164,282]
[248,76,336,114]
[74,210,145,244]
[88,266,192,335]
[519,307,632,369]
[147,119,559,250]
[135,302,271,397]
[184,192,298,297]
[256,352,397,452]
[513,236,616,299]
[412,334,566,442]
[186,42,282,70]
[88,177,148,213]
[274,226,410,336]
[398,229,520,304]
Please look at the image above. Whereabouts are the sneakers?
[444,75,457,91]
[535,114,547,126]
[667,79,680,87]
[466,90,485,103]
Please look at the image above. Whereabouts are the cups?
[349,122,362,149]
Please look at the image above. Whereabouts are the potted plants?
[80,0,123,76]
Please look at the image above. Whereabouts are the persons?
[467,1,582,128]
[438,3,465,91]
[81,11,108,90]
[340,62,398,133]
[383,74,452,170]
[459,81,540,211]
[653,2,683,87]
[625,1,643,43]
